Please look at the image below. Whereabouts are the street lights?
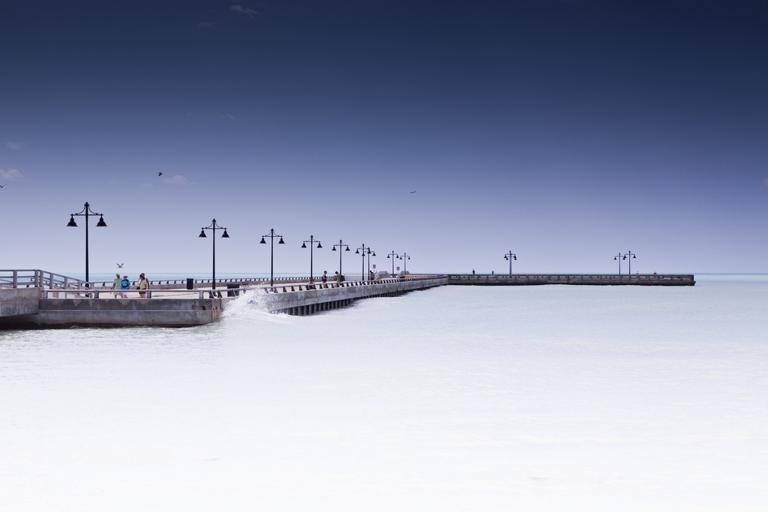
[301,233,411,289]
[502,249,517,278]
[613,252,626,276]
[623,250,636,278]
[65,201,107,297]
[259,226,286,293]
[199,217,229,296]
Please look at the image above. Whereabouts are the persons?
[322,270,329,288]
[111,273,124,300]
[137,274,147,298]
[369,270,374,281]
[119,274,130,298]
[138,272,150,289]
[334,271,340,287]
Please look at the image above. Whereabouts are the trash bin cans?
[187,279,193,289]
[400,276,405,282]
[341,276,345,281]
[226,284,239,296]
